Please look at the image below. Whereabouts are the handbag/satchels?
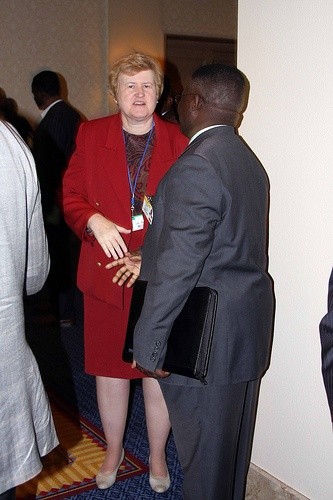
[122,279,218,386]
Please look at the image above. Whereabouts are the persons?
[0,119,60,500]
[62,52,190,492]
[0,70,83,328]
[156,88,179,124]
[106,62,276,500]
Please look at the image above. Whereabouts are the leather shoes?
[96,448,124,489]
[148,456,171,492]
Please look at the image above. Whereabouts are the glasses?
[175,94,206,106]
